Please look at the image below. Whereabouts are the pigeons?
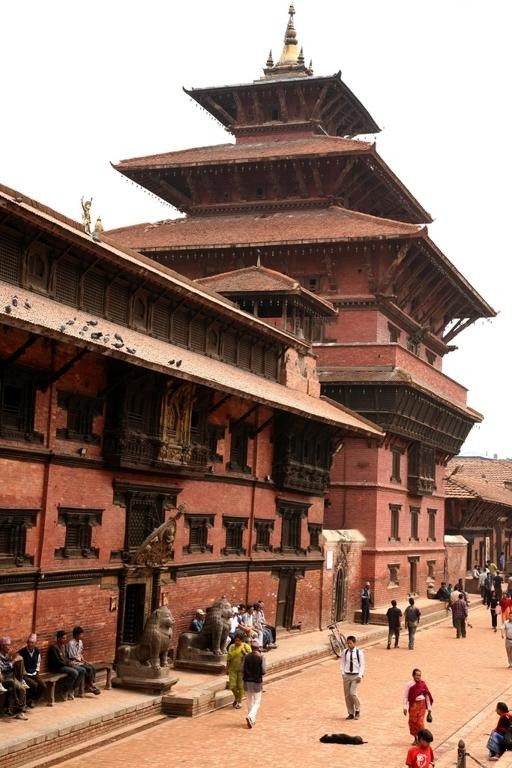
[59,317,136,355]
[6,295,31,313]
[93,234,101,243]
[168,359,182,366]
[13,196,24,203]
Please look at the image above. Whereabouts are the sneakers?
[346,711,359,720]
[246,716,253,728]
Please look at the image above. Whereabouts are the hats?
[196,609,207,615]
[232,607,239,614]
[28,633,37,642]
[0,636,11,645]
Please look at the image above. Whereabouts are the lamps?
[265,475,270,481]
[79,448,88,454]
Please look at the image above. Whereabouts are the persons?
[406,729,434,768]
[191,600,277,728]
[360,582,371,624]
[403,669,432,745]
[501,612,512,670]
[341,636,364,719]
[386,600,402,649]
[94,215,104,233]
[404,598,421,650]
[486,703,512,760]
[436,579,470,637]
[80,194,93,234]
[473,552,512,631]
[0,627,100,720]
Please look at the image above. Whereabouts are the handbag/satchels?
[427,715,432,722]
[14,661,25,680]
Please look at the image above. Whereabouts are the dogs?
[320,733,364,745]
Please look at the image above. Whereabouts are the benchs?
[0,661,114,707]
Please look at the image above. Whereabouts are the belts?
[346,673,358,675]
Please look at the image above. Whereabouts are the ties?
[350,651,353,672]
